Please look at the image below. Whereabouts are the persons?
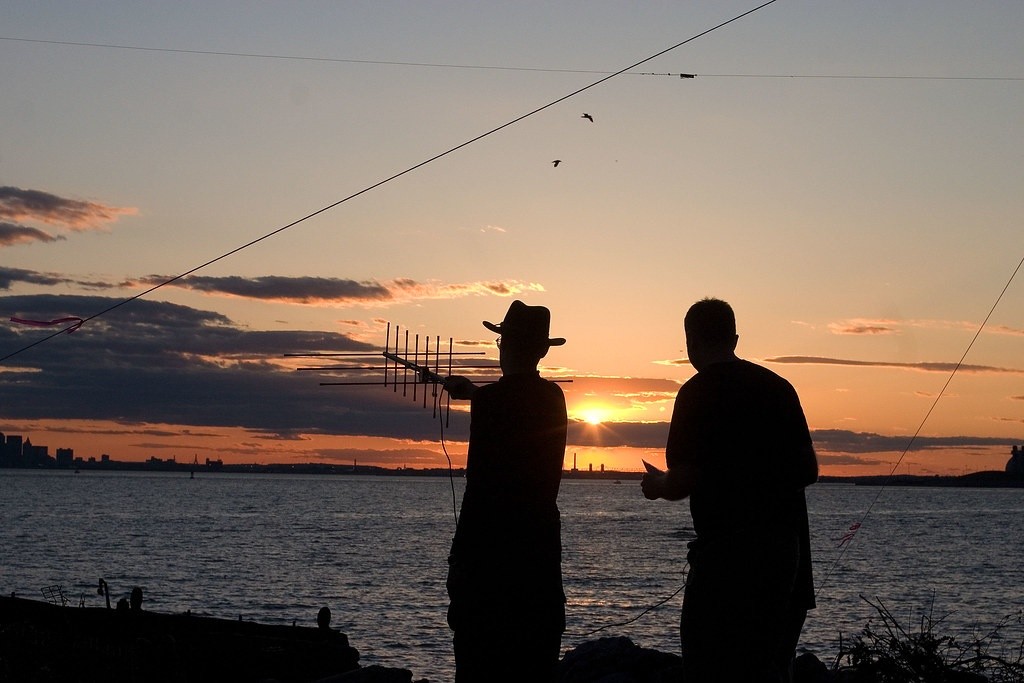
[641,298,818,683]
[443,301,569,683]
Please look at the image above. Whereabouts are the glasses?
[496,336,506,349]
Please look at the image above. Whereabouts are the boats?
[613,480,621,483]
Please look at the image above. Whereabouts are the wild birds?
[551,112,593,167]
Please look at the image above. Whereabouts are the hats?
[483,300,566,346]
